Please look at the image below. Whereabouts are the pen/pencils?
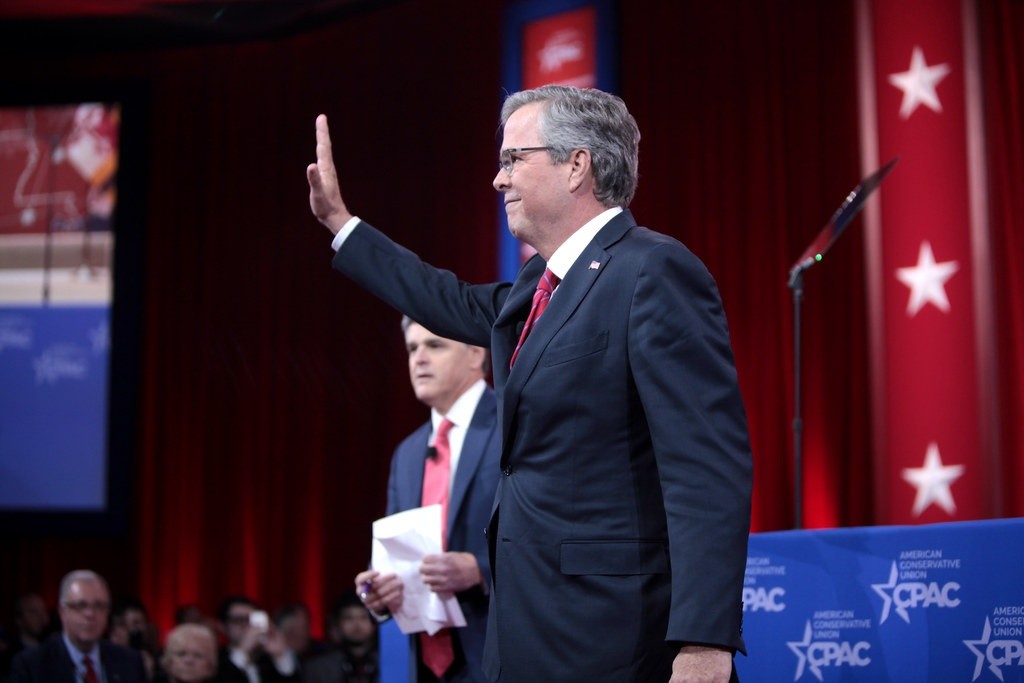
[361,580,370,599]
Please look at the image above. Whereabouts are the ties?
[82,656,100,683]
[419,419,455,678]
[510,267,561,372]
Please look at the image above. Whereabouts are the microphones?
[428,447,436,459]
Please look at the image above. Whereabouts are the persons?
[0,569,379,683]
[308,82,755,683]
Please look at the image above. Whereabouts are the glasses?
[227,615,249,624]
[63,600,109,612]
[499,146,559,174]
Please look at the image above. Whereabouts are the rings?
[361,592,369,605]
